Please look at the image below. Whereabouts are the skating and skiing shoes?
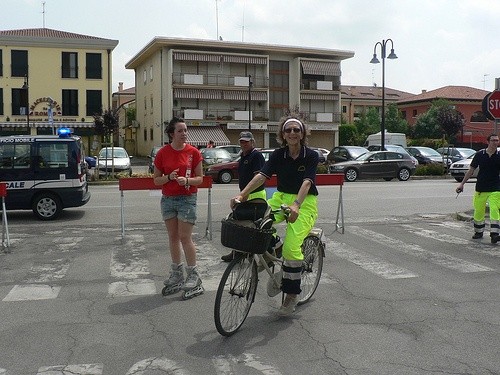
[162,262,186,296]
[180,264,205,300]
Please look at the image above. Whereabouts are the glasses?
[283,128,301,133]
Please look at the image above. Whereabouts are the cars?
[312,144,478,166]
[146,146,163,174]
[94,146,134,179]
[84,156,96,169]
[198,145,276,183]
[328,150,416,184]
[449,147,500,182]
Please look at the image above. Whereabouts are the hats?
[239,131,254,142]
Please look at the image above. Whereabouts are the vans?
[0,127,91,221]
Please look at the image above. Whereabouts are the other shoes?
[257,253,281,272]
[491,235,500,243]
[472,231,483,239]
[221,250,247,262]
[277,294,299,317]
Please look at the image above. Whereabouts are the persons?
[221,116,319,315]
[456,134,500,243]
[154,117,207,300]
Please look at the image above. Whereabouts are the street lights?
[22,74,30,134]
[248,75,254,132]
[369,38,398,150]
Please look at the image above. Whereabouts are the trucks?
[363,132,407,149]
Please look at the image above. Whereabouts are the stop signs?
[486,89,500,121]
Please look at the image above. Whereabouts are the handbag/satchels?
[226,198,272,229]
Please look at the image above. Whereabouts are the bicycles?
[213,206,326,337]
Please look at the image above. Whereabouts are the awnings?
[0,123,97,128]
[184,127,230,146]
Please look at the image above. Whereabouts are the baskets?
[221,217,277,254]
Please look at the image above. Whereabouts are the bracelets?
[293,200,301,209]
[186,178,188,185]
[168,173,171,181]
